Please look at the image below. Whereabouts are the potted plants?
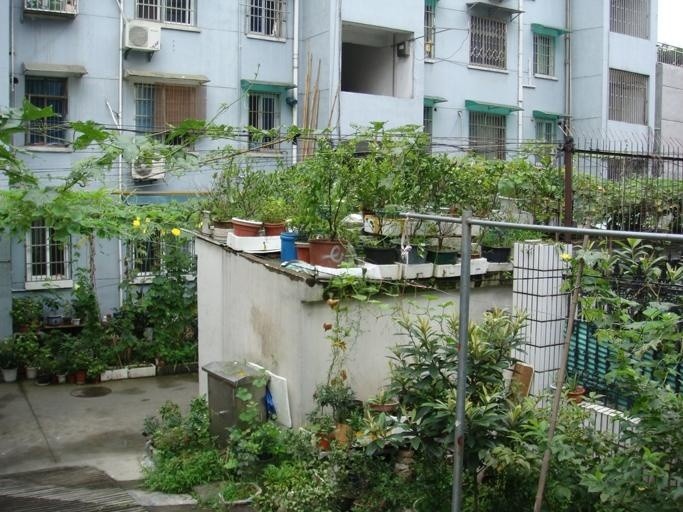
[193,115,617,266]
[0,274,196,387]
[214,368,400,511]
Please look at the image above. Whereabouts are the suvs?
[279,231,310,262]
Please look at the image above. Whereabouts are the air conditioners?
[122,19,162,55]
[128,151,167,183]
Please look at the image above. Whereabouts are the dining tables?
[214,368,400,511]
[0,274,196,387]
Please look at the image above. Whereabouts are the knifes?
[122,19,162,55]
[128,151,167,183]
